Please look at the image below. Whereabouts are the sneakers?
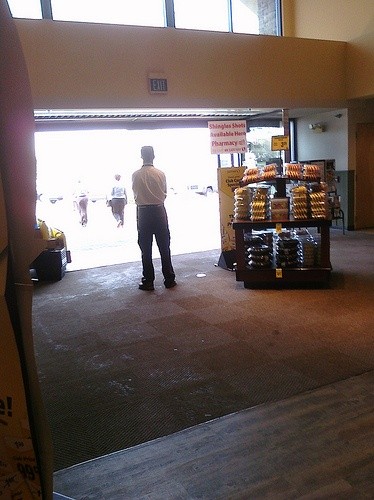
[164,278,176,288]
[138,280,154,291]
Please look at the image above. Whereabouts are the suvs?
[165,153,256,197]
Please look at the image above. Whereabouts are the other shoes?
[117,220,122,228]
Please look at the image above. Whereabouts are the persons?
[75,180,88,227]
[110,175,127,227]
[132,146,176,290]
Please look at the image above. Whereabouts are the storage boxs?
[36,246,67,281]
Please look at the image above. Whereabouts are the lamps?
[309,123,325,132]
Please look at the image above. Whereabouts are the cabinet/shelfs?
[233,177,334,289]
